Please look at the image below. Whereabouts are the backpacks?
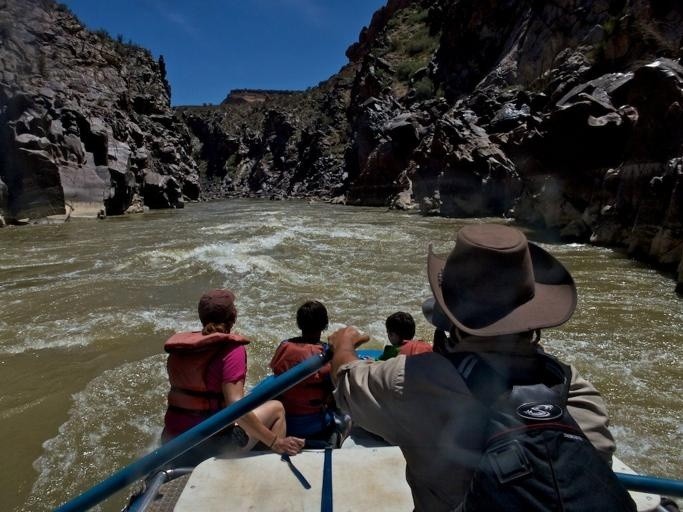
[432,350,634,512]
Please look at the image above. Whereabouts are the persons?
[156,288,306,461]
[371,308,428,357]
[325,222,616,510]
[269,297,352,449]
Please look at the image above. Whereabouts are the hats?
[198,289,236,324]
[427,224,577,337]
